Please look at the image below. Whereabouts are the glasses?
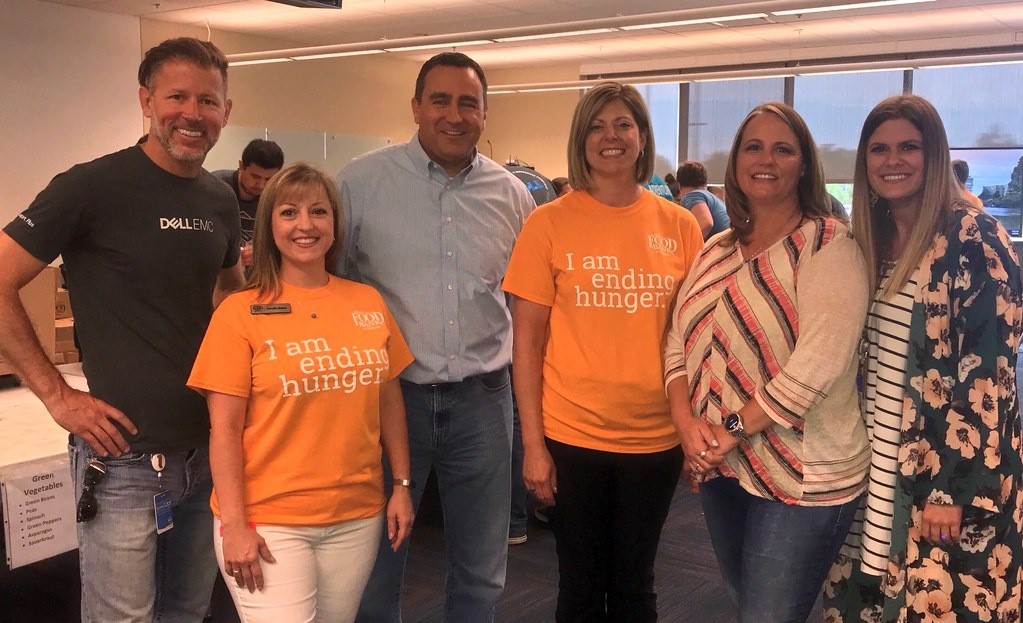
[75,456,108,522]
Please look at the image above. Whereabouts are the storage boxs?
[0,266,79,375]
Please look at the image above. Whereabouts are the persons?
[846,96,1023,623]
[664,101,871,623]
[208,138,983,292]
[186,164,417,623]
[501,80,705,623]
[0,38,249,623]
[325,53,537,623]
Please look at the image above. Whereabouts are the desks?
[0,360,110,486]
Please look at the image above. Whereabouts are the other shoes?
[532,507,552,529]
[508,527,527,544]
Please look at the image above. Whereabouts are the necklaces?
[888,254,900,269]
[749,207,802,261]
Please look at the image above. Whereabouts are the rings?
[693,463,700,475]
[231,568,239,574]
[699,450,707,458]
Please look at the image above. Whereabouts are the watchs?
[392,477,414,488]
[724,411,751,442]
